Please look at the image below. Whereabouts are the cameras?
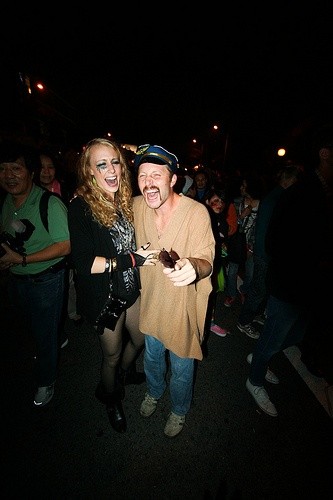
[0,218,36,258]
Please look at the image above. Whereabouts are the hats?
[180,174,193,195]
[132,143,184,176]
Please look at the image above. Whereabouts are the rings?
[141,246,144,250]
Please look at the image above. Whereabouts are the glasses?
[157,247,177,268]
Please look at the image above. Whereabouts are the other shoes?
[33,377,57,407]
[210,324,226,337]
[239,293,248,305]
[237,320,260,339]
[222,292,241,307]
[252,313,267,326]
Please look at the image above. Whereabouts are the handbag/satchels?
[113,267,140,310]
[95,287,128,331]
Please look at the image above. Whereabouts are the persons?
[236,166,302,340]
[247,118,333,417]
[0,144,71,405]
[130,143,214,437]
[32,149,62,197]
[193,170,210,203]
[200,189,227,338]
[223,173,251,308]
[67,138,146,434]
[242,177,266,290]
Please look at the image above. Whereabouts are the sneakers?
[246,377,279,416]
[246,353,280,384]
[138,390,161,417]
[163,411,186,437]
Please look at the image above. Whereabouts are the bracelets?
[104,256,117,273]
[22,254,27,267]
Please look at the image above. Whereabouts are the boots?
[116,358,146,386]
[94,376,126,432]
